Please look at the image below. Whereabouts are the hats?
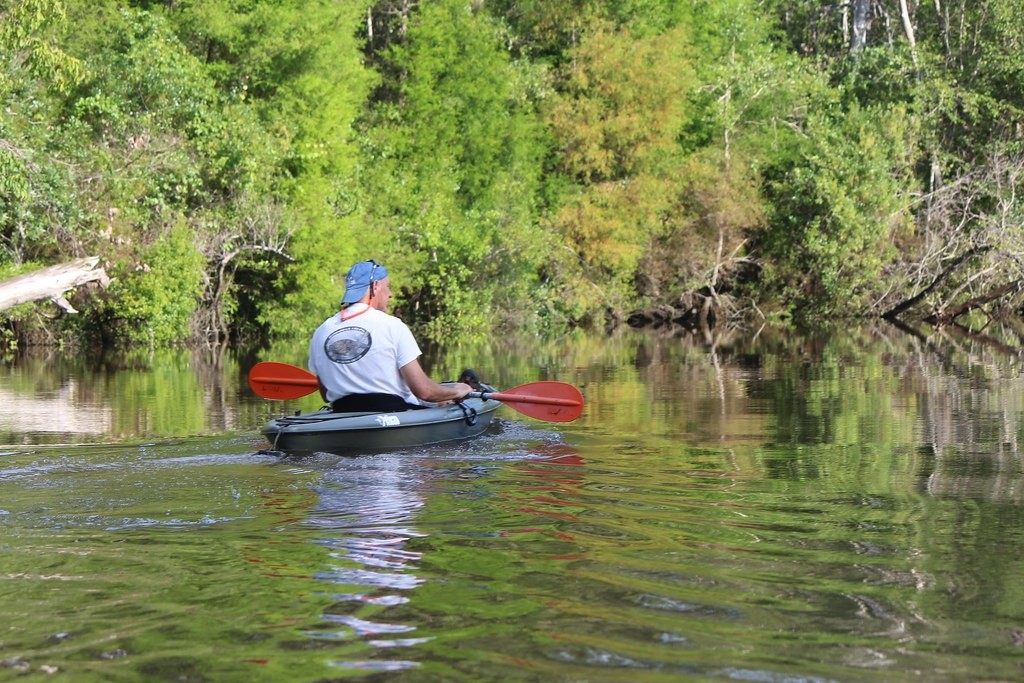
[341,262,388,305]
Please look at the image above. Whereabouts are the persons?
[306,259,476,413]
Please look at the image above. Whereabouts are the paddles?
[247,361,584,424]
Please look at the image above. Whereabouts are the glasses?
[367,260,379,299]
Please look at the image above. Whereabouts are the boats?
[258,379,504,459]
